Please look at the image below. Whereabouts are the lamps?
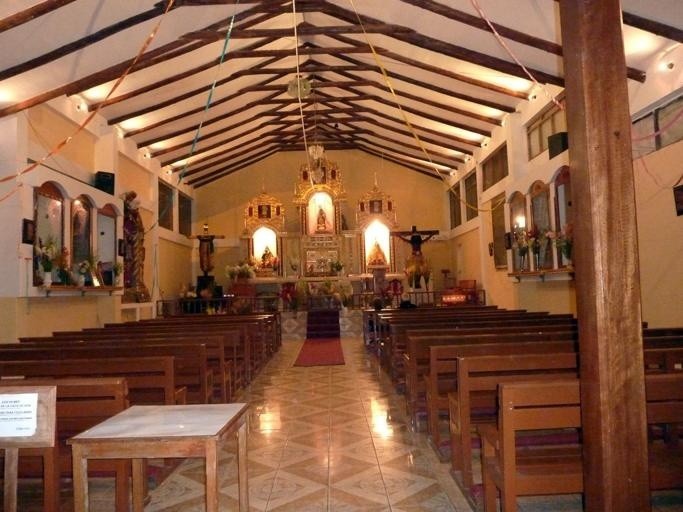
[288,73,312,99]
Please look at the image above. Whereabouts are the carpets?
[294,306,345,365]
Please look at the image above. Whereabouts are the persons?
[367,241,387,266]
[260,247,276,267]
[314,207,333,234]
[396,233,437,288]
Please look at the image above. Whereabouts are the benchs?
[359,305,682,510]
[0,311,281,511]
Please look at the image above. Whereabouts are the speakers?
[95,171,114,196]
[547,132,569,160]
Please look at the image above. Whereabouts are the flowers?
[57,247,126,285]
[226,264,257,277]
[515,224,576,259]
[37,236,58,272]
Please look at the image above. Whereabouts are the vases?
[43,271,52,286]
[519,254,572,269]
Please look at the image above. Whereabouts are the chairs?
[458,280,476,290]
[442,269,456,288]
